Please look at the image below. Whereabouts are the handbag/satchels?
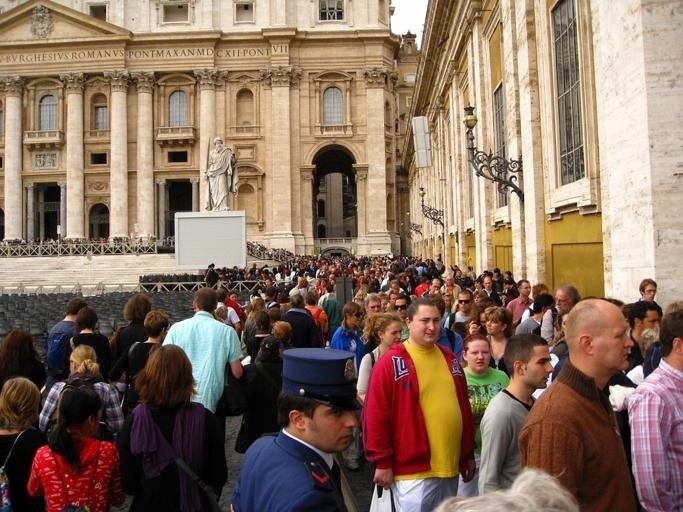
[121,389,138,417]
[0,466,12,512]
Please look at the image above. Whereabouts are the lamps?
[462,99,525,201]
[398,186,445,239]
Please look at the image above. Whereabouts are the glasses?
[395,306,407,310]
[458,300,469,304]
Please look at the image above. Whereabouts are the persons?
[518,298,636,508]
[205,255,682,471]
[247,241,293,260]
[114,345,228,507]
[27,384,125,507]
[3,377,48,507]
[161,287,243,415]
[438,466,578,508]
[3,236,175,253]
[2,294,172,441]
[228,349,363,507]
[628,312,682,506]
[477,333,555,494]
[202,137,236,211]
[456,333,510,498]
[361,297,477,508]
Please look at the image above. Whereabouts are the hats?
[282,348,364,410]
